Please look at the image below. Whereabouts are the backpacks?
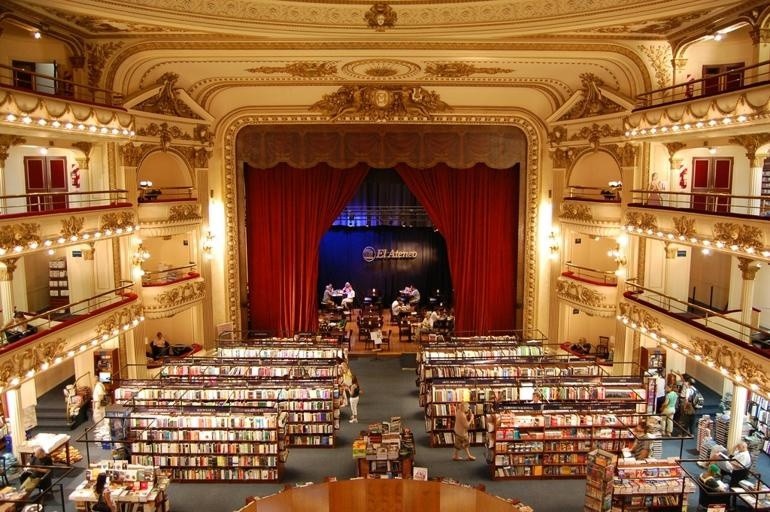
[690,387,704,409]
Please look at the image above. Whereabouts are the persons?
[153,331,166,347]
[322,284,334,305]
[392,297,404,315]
[730,442,752,487]
[411,311,433,340]
[18,447,53,489]
[339,362,359,423]
[409,284,420,304]
[335,314,346,331]
[1,312,38,342]
[341,282,355,309]
[700,464,731,507]
[431,306,455,328]
[319,318,331,337]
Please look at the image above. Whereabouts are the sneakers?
[662,431,694,439]
[452,456,477,461]
[348,415,358,424]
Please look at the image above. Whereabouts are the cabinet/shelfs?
[158,355,348,409]
[352,430,414,480]
[215,335,348,359]
[481,397,663,481]
[69,463,172,512]
[424,374,658,448]
[582,448,696,511]
[746,392,770,457]
[418,356,600,409]
[641,346,666,386]
[415,338,545,388]
[113,378,342,449]
[104,404,291,484]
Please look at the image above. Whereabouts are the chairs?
[0,454,53,511]
[319,290,455,352]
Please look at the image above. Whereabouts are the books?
[352,416,415,479]
[76,335,349,511]
[731,483,770,508]
[697,393,770,463]
[707,503,727,512]
[417,334,696,512]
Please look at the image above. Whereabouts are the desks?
[18,432,71,469]
